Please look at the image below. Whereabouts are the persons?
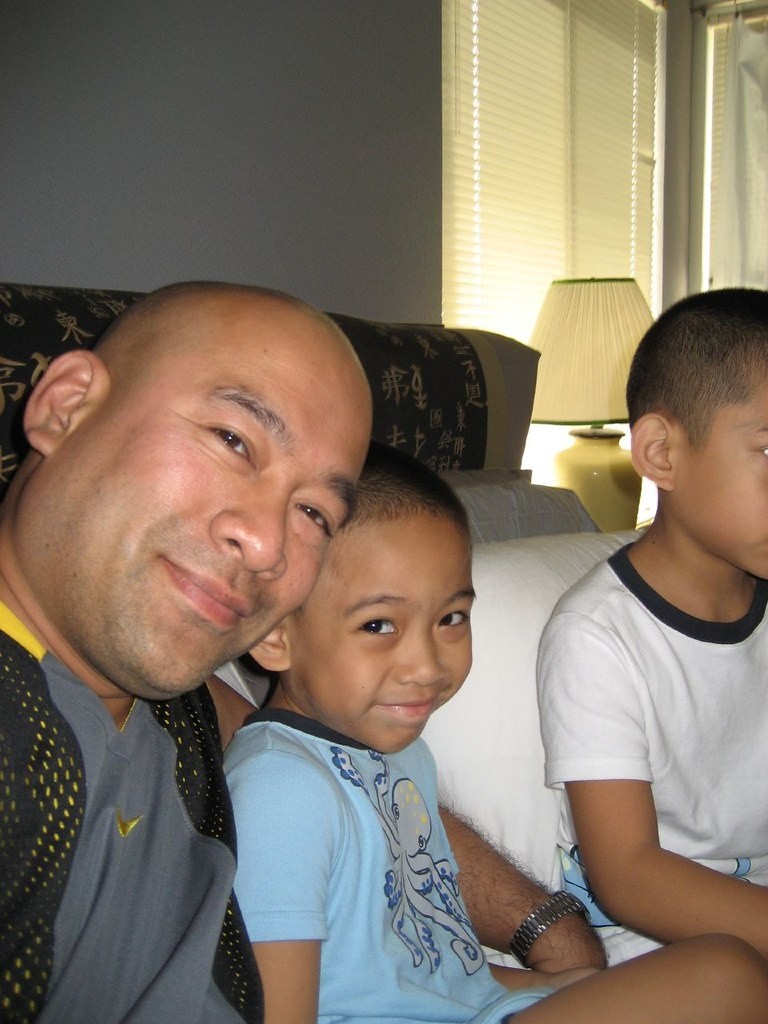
[223,430,768,1024]
[537,286,765,968]
[2,280,608,1023]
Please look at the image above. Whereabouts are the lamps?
[522,275,656,534]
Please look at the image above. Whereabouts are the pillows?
[210,478,647,966]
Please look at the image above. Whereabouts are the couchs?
[3,278,544,698]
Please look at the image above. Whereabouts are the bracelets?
[509,889,592,968]
